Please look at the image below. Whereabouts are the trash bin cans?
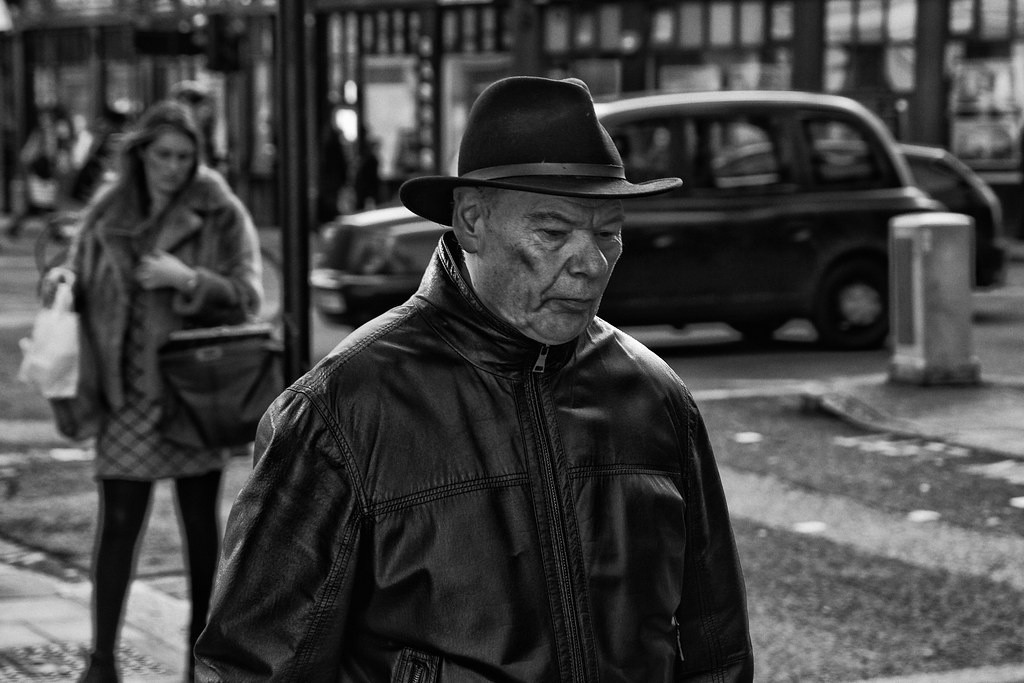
[890,210,984,386]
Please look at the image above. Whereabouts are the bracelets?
[184,267,198,295]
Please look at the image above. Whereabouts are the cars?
[310,91,947,357]
[713,138,1007,294]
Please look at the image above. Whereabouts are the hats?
[399,77,683,227]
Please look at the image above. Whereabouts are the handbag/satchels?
[18,283,78,400]
[158,277,285,450]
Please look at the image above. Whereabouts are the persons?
[0,80,237,242]
[194,76,754,683]
[36,108,265,683]
